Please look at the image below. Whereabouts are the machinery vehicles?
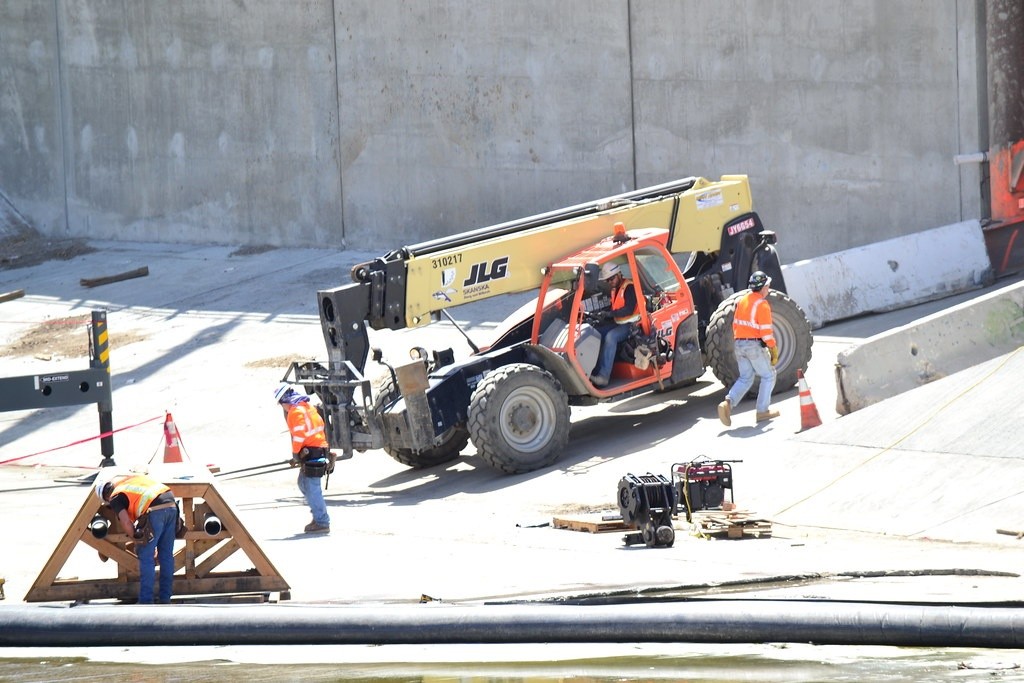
[276,172,813,478]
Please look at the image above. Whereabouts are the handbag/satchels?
[133,515,155,544]
[305,459,326,477]
[176,516,187,539]
[326,452,336,474]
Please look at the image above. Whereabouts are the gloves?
[591,310,612,319]
[770,346,778,365]
[293,453,299,463]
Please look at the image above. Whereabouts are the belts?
[735,338,762,340]
[147,502,176,513]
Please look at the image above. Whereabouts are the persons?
[272,382,330,533]
[718,271,781,426]
[583,261,646,387]
[94,473,178,604]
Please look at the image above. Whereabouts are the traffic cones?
[161,412,184,464]
[796,367,823,434]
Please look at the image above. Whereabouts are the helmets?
[274,382,291,404]
[598,262,621,281]
[749,271,772,287]
[95,481,111,502]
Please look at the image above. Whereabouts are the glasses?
[607,274,618,282]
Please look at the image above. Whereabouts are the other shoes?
[304,519,330,533]
[757,410,779,422]
[718,400,731,426]
[588,374,609,388]
